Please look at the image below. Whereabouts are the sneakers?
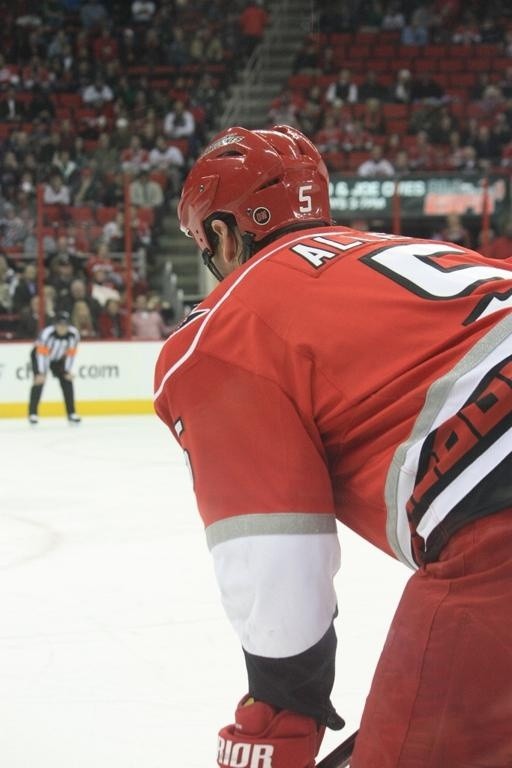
[28,412,82,424]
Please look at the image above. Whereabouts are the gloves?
[215,691,326,768]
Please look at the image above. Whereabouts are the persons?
[27,310,83,422]
[148,122,510,768]
[1,1,511,340]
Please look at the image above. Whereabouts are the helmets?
[176,123,333,284]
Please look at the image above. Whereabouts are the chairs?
[0,0,511,342]
